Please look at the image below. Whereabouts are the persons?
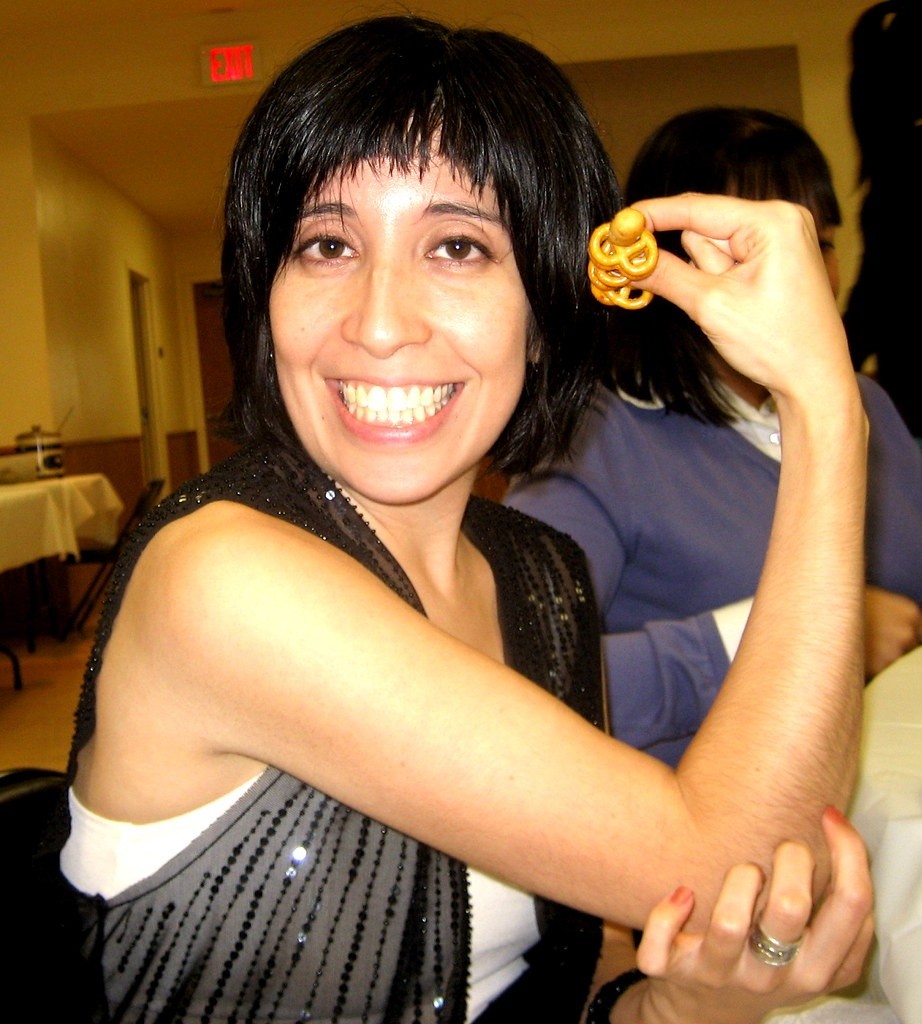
[54,15,874,1024]
[502,106,922,772]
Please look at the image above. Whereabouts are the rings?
[753,923,803,967]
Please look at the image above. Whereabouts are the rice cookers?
[15,424,66,479]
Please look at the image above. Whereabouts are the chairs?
[1,767,107,1024]
[55,479,165,643]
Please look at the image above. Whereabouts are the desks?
[0,474,125,691]
